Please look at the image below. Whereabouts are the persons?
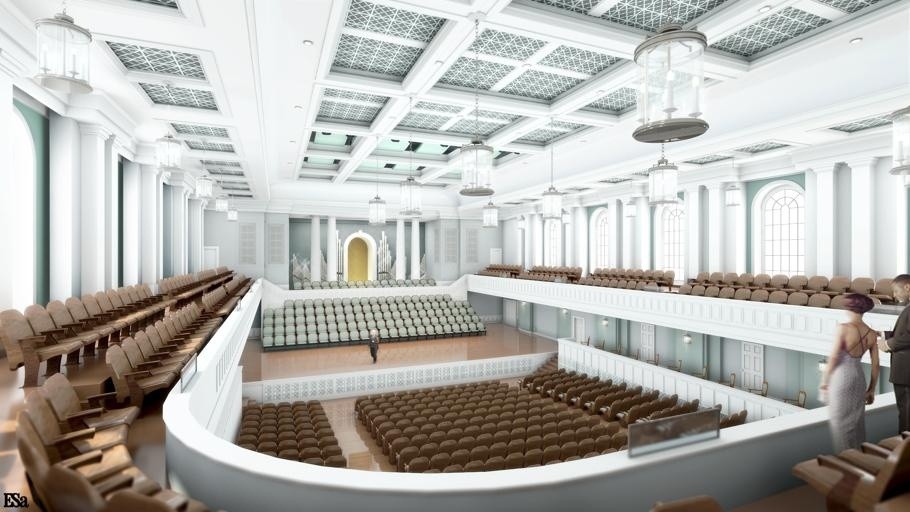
[819,293,881,452]
[368,333,379,363]
[880,274,909,431]
